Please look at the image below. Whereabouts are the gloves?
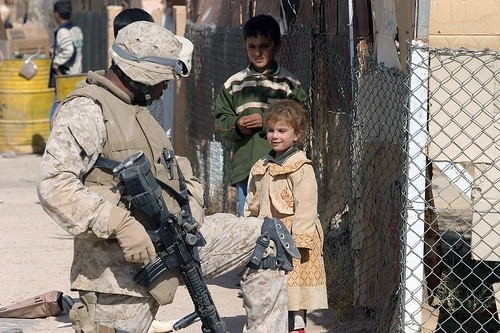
[107,204,157,263]
[188,196,205,229]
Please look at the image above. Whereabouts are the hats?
[109,20,181,86]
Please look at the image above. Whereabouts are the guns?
[111,151,224,333]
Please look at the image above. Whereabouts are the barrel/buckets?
[0,58,53,154]
[55,70,105,104]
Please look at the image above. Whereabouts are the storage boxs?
[0,25,50,59]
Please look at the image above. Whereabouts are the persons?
[115,8,163,128]
[243,100,329,333]
[215,15,307,218]
[0,20,302,333]
[0,0,11,58]
[46,0,83,120]
